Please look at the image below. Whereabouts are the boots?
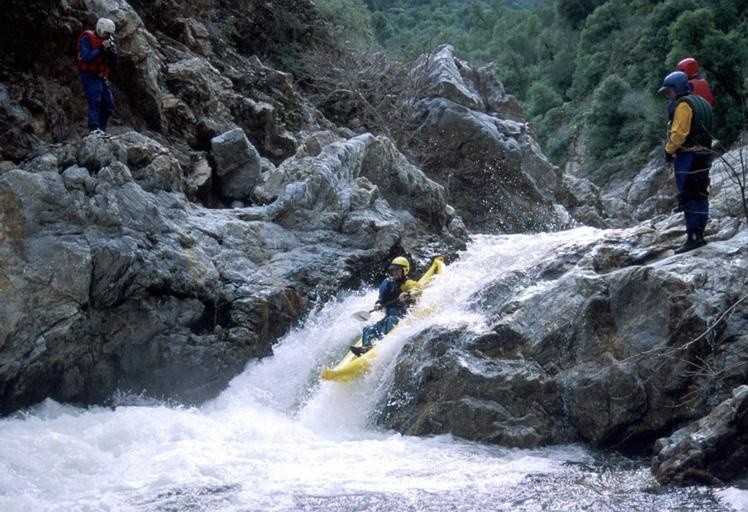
[674,229,706,255]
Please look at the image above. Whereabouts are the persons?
[350,256,421,357]
[658,70,714,254]
[675,58,713,106]
[75,17,117,132]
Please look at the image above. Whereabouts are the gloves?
[103,39,118,55]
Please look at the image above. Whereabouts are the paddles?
[352,287,422,321]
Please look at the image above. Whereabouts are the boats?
[321,257,447,383]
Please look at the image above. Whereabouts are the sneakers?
[89,128,111,138]
[350,346,368,357]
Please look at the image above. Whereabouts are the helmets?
[677,58,698,78]
[387,257,409,280]
[96,18,116,38]
[657,72,691,97]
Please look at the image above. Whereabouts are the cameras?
[104,35,118,54]
